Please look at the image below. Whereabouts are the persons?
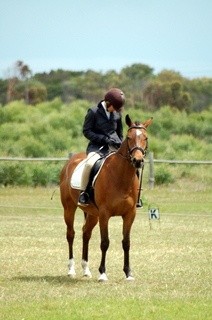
[79,87,143,208]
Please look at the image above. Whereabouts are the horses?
[59,114,153,283]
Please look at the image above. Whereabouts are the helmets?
[105,88,125,112]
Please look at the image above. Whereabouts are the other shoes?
[78,192,89,205]
